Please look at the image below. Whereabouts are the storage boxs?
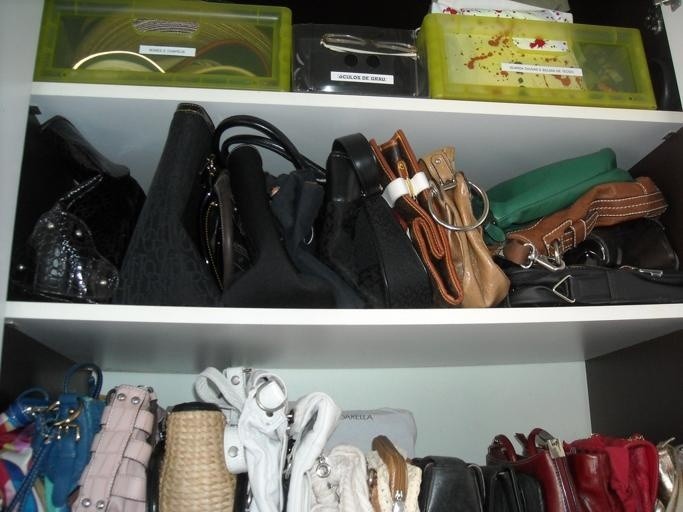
[31,0,296,92]
[420,13,658,109]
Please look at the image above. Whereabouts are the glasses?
[320,33,417,57]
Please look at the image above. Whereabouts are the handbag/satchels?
[8,102,683,309]
[0,362,683,512]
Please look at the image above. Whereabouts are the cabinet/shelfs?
[1,84,683,371]
